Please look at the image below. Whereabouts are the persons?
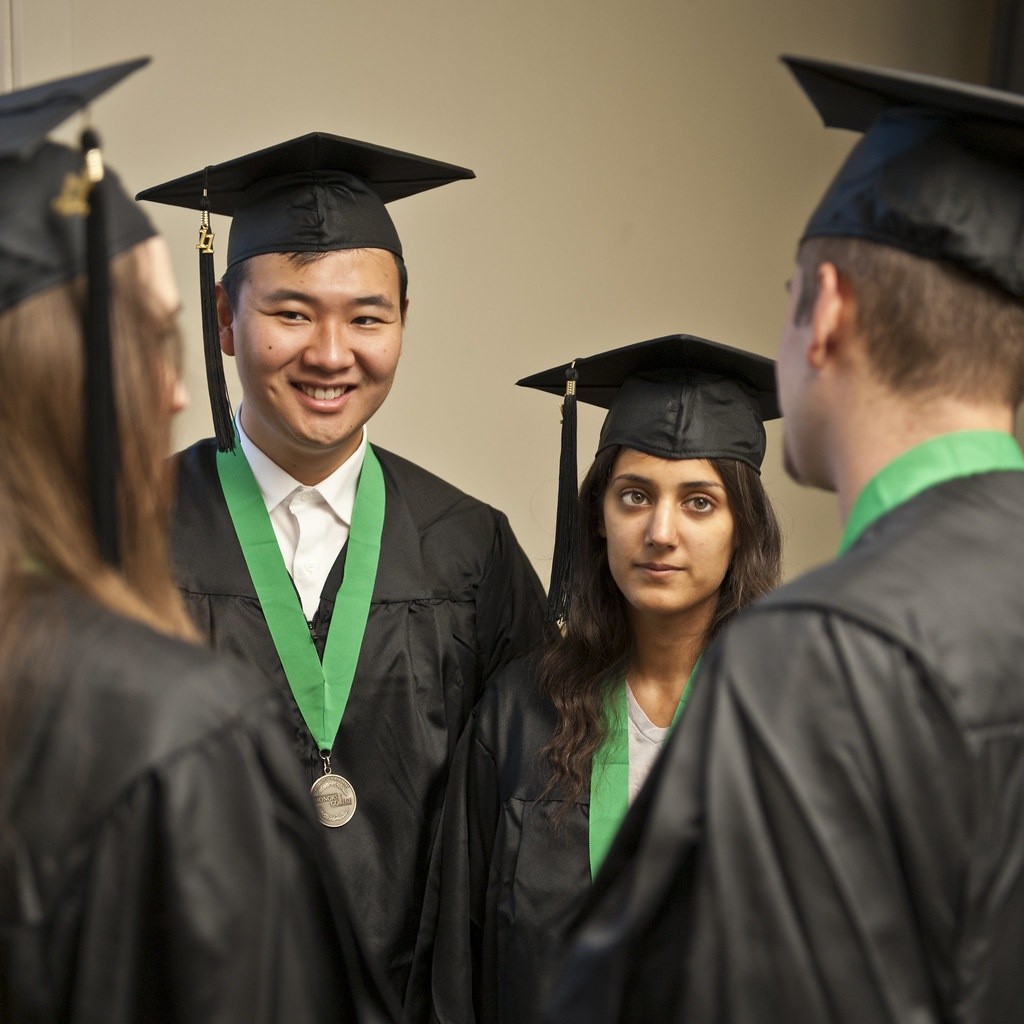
[417,332,784,1023]
[1,54,389,1024]
[547,44,1023,1024]
[133,126,563,1023]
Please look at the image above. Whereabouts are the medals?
[310,771,360,832]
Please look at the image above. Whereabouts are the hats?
[0,55,159,566]
[516,335,785,619]
[136,132,475,453]
[768,54,1024,309]
